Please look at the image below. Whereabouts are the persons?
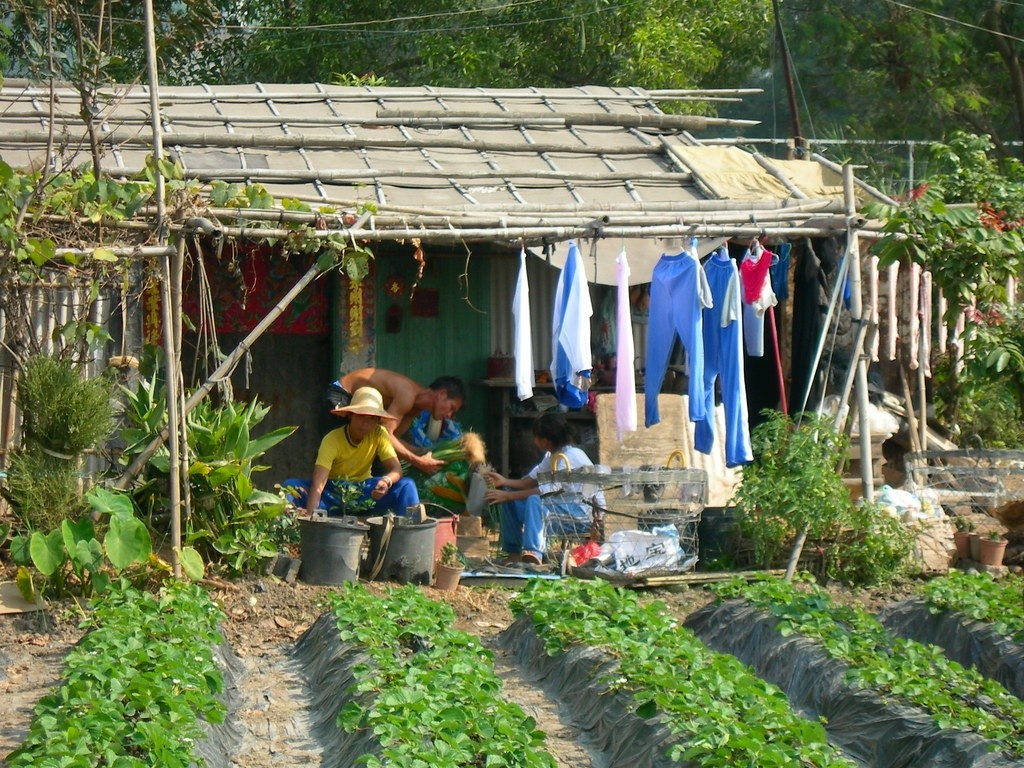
[278,387,421,517]
[483,411,605,565]
[325,367,465,476]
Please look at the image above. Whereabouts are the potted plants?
[980,530,1008,565]
[435,541,465,591]
[954,516,977,558]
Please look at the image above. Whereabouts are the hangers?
[520,223,791,267]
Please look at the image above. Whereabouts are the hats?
[331,387,398,420]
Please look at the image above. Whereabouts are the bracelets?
[382,476,392,489]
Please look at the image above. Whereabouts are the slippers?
[492,558,506,567]
[521,554,542,567]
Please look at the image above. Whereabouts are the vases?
[970,532,980,558]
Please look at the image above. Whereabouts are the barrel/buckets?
[416,502,463,575]
[297,509,372,587]
[696,506,754,574]
[637,508,698,553]
[366,510,439,585]
